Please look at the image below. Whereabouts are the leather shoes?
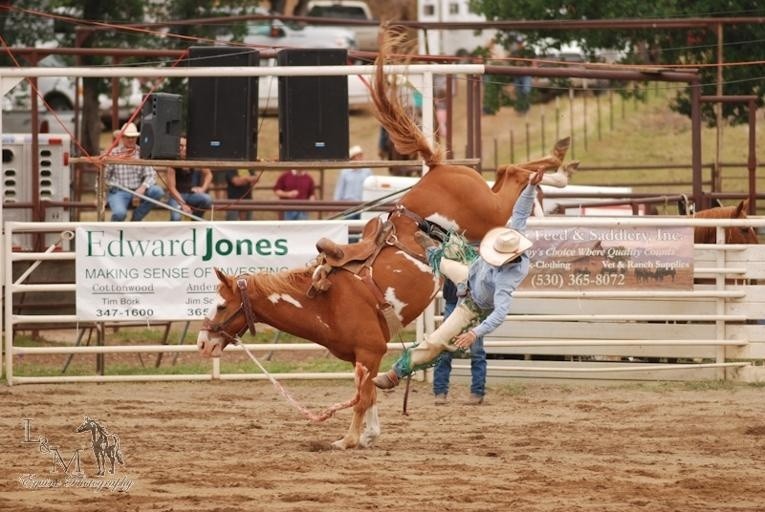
[433,392,447,404]
[462,393,484,405]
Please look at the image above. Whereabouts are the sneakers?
[413,231,436,251]
[371,370,401,390]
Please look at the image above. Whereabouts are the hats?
[348,145,363,159]
[479,227,533,268]
[113,122,141,138]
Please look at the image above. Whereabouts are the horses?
[647,198,759,324]
[196,12,581,451]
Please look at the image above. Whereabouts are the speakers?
[276,47,350,162]
[186,46,260,162]
[139,92,183,160]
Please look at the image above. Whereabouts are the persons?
[163,134,214,222]
[430,276,489,406]
[332,144,375,246]
[223,168,261,222]
[273,168,317,222]
[368,164,544,389]
[102,119,165,221]
[377,120,421,178]
[502,35,535,115]
